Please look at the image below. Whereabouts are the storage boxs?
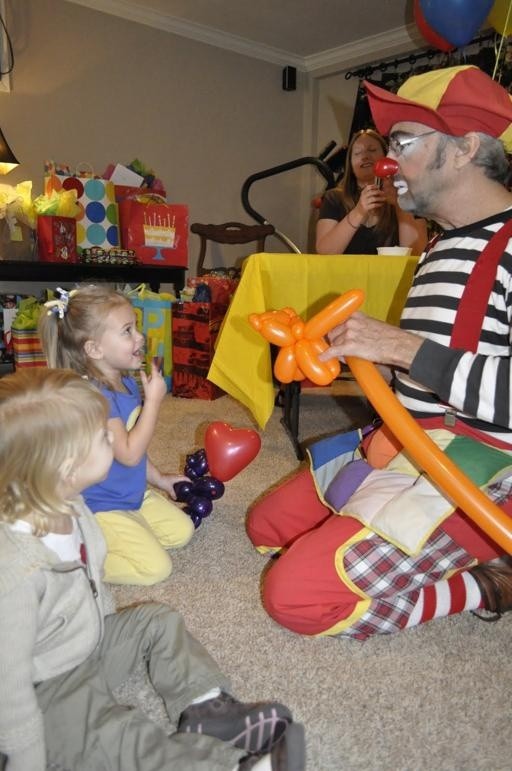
[172,302,231,400]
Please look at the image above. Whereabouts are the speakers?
[281,65,298,91]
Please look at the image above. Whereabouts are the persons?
[243,64,512,641]
[2,364,305,771]
[309,126,433,256]
[32,282,197,588]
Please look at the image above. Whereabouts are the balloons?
[411,0,512,53]
[166,417,265,531]
[246,287,512,556]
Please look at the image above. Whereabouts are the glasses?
[390,130,436,155]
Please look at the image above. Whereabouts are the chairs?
[191,221,274,277]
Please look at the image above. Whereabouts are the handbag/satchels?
[129,299,227,401]
[43,174,189,268]
[11,326,48,371]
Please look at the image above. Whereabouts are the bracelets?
[346,214,359,231]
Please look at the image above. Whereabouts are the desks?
[206,253,424,463]
[0,260,188,299]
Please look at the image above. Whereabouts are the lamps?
[0,126,20,175]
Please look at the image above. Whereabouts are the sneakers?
[468,558,512,614]
[173,693,306,770]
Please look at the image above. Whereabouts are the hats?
[362,64,511,155]
